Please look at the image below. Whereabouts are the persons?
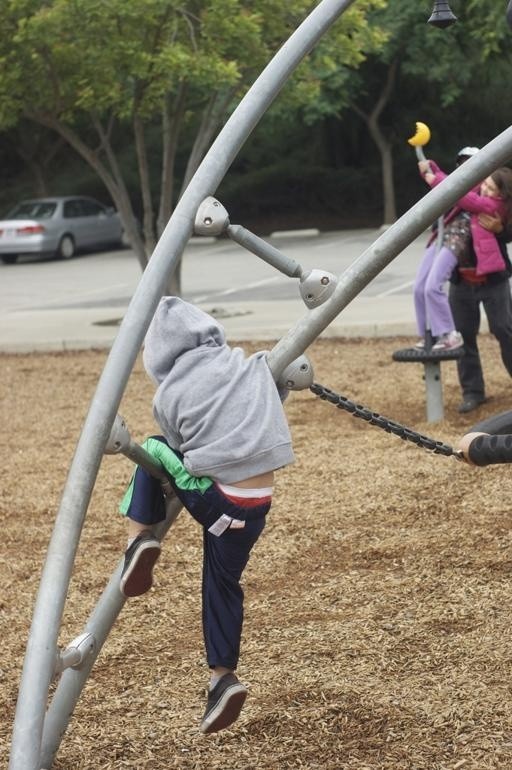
[119,295,296,735]
[413,146,511,415]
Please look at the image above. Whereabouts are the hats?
[458,147,480,155]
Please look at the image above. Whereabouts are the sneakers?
[197,673,246,735]
[430,332,463,353]
[413,340,424,350]
[119,531,160,598]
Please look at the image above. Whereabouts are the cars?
[0,195,140,266]
